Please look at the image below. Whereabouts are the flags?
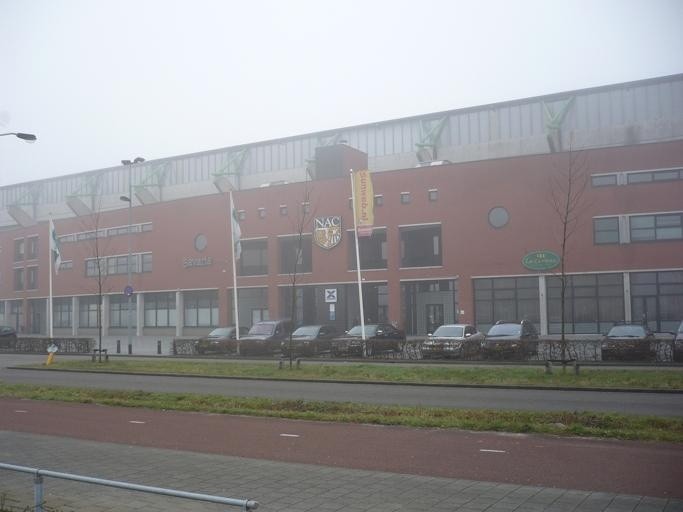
[352,167,375,227]
[230,198,242,259]
[48,217,61,275]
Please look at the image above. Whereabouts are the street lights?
[120,196,132,354]
[1,132,35,141]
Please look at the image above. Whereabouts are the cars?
[601,324,650,361]
[195,327,249,354]
[330,323,405,356]
[670,321,683,362]
[482,320,538,360]
[0,326,16,349]
[420,324,485,358]
[281,325,337,351]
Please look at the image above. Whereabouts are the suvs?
[240,321,289,354]
